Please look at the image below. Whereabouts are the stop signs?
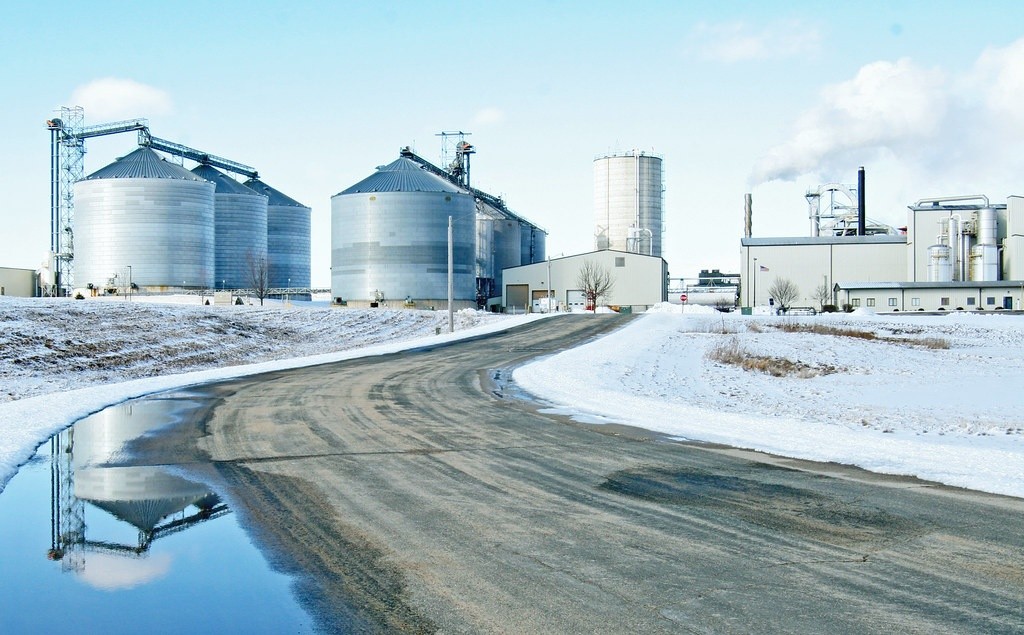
[680,295,686,301]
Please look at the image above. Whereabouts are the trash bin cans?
[741,307,752,315]
[619,307,632,313]
[491,304,500,312]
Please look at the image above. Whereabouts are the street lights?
[548,252,565,313]
[754,257,757,307]
[127,266,132,301]
